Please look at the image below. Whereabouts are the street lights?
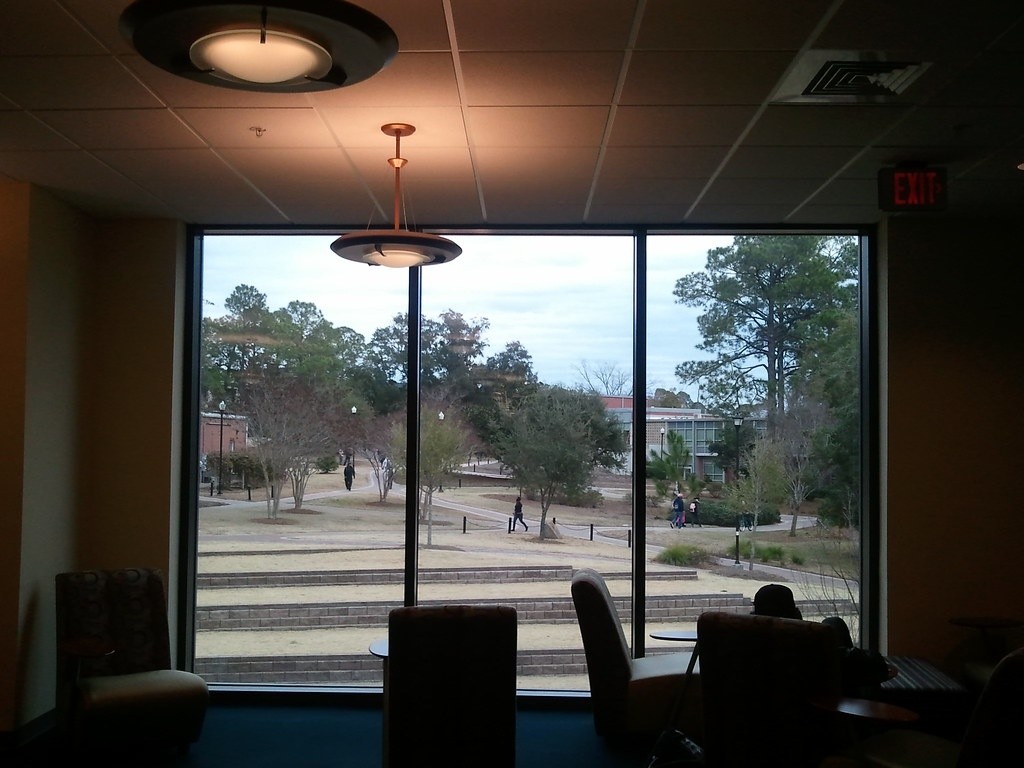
[659,428,665,460]
[217,401,228,494]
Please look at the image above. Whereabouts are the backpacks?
[689,502,696,513]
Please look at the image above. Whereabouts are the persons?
[510,496,530,535]
[669,492,705,530]
[343,461,357,492]
[738,499,754,528]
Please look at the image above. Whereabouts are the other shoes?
[670,522,675,528]
[511,528,515,531]
[676,523,678,526]
[682,523,686,527]
[526,526,528,531]
[679,527,683,530]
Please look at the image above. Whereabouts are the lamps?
[113,1,403,99]
[330,121,462,270]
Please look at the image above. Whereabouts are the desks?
[948,617,1024,664]
[809,696,918,768]
[648,634,699,768]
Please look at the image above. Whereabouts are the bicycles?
[738,512,756,531]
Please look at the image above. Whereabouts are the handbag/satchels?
[646,728,705,768]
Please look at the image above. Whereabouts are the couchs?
[54,566,212,759]
[853,646,1024,768]
[380,603,518,768]
[696,609,861,767]
[571,567,701,740]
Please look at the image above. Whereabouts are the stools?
[879,656,968,720]
